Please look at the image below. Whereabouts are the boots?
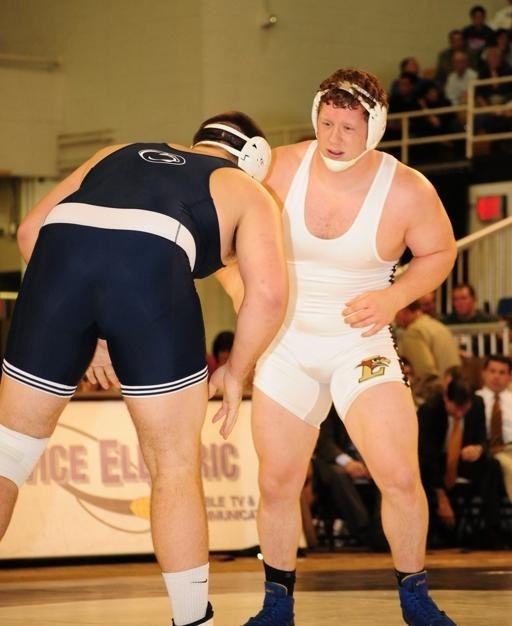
[243,582,295,626]
[399,570,457,626]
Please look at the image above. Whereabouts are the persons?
[211,330,254,402]
[214,67,463,626]
[300,289,512,554]
[2,109,295,626]
[377,4,512,312]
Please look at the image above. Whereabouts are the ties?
[444,417,463,489]
[488,394,505,454]
